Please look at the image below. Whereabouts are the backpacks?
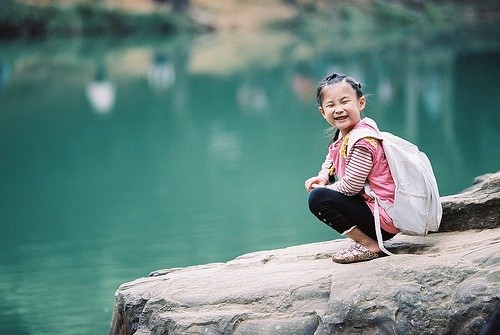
[346,118,442,236]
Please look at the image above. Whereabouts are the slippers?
[332,243,384,263]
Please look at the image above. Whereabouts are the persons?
[304,72,403,264]
